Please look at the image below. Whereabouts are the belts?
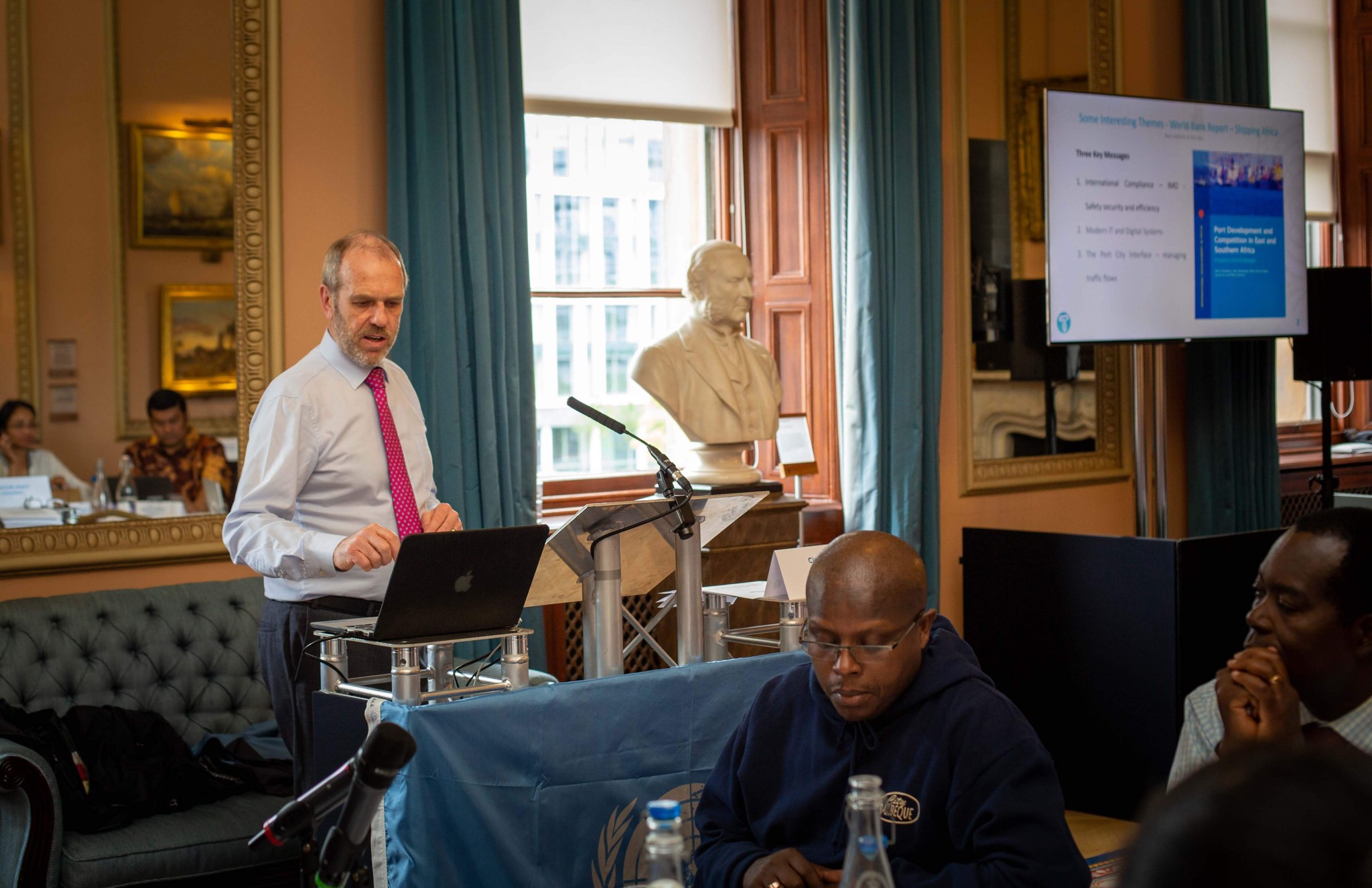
[273,594,383,618]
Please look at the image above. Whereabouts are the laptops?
[108,475,171,507]
[311,524,551,641]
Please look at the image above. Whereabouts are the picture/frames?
[122,120,236,251]
[156,284,239,397]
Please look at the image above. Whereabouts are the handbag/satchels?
[0,695,97,835]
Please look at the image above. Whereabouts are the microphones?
[247,722,417,888]
[567,396,695,495]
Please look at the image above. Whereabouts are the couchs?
[0,577,364,888]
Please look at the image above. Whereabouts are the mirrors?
[951,0,1134,497]
[0,0,286,574]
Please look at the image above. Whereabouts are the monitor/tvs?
[1039,86,1309,345]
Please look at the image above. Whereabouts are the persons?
[0,399,94,510]
[679,530,1094,888]
[1111,718,1372,888]
[630,241,782,484]
[1159,511,1369,792]
[221,230,467,888]
[120,388,234,516]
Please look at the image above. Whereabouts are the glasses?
[798,607,926,664]
[10,422,41,430]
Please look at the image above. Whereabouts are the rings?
[1270,672,1280,683]
[768,882,780,888]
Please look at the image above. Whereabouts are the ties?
[363,367,426,542]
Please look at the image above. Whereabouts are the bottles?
[93,458,109,519]
[641,799,685,887]
[116,455,137,514]
[837,774,895,888]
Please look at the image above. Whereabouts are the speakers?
[1292,265,1372,382]
[1010,279,1079,384]
